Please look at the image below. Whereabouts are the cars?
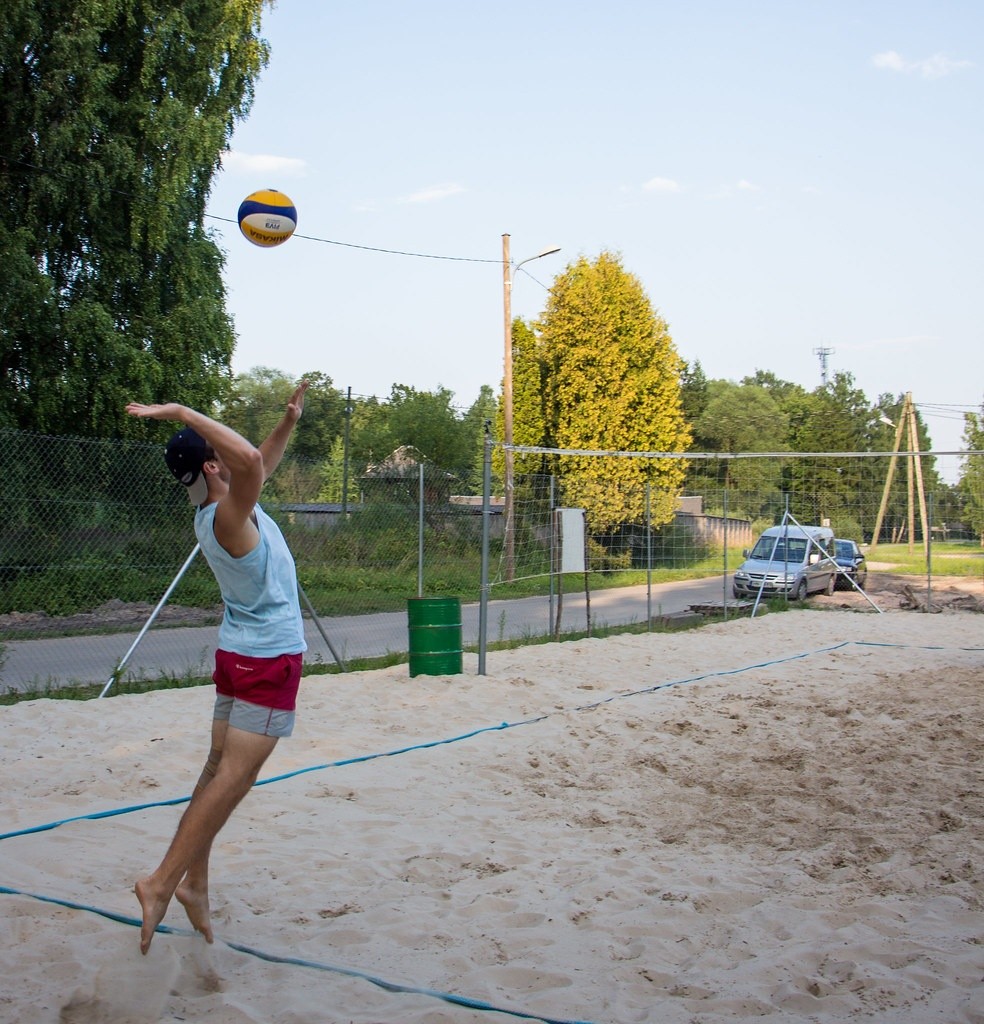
[834,538,867,592]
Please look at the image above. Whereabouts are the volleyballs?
[237,189,299,249]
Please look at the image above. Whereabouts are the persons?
[126,381,310,955]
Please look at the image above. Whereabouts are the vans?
[733,526,837,602]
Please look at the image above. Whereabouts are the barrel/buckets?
[407,597,464,678]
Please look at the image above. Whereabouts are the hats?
[164,428,209,506]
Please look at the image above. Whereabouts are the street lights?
[500,232,562,584]
